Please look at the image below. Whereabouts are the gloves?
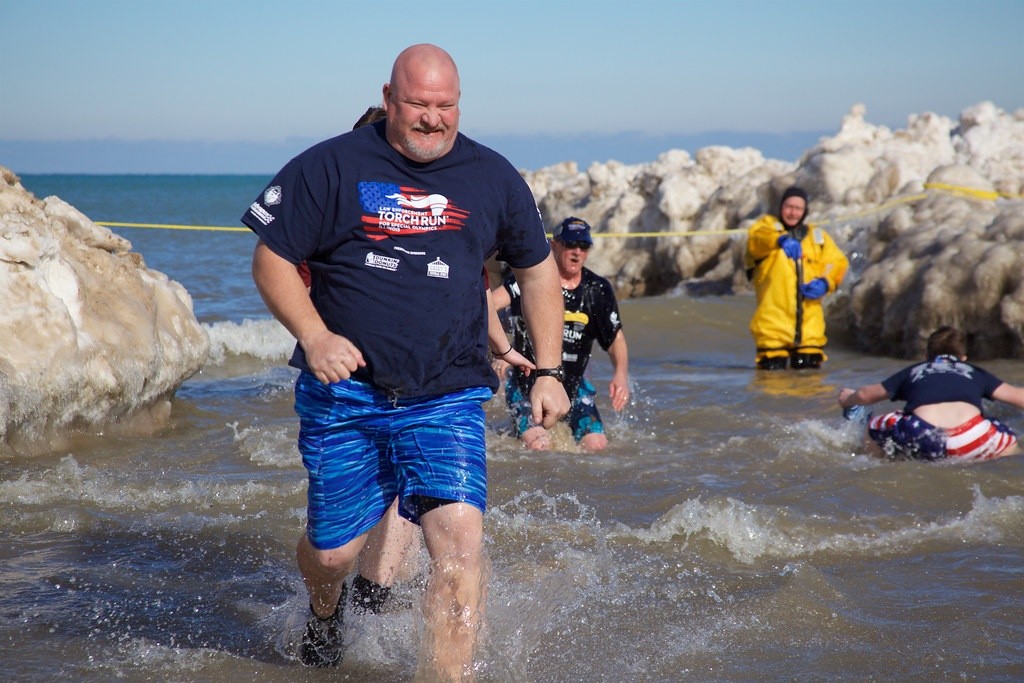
[779,234,802,260]
[800,279,828,299]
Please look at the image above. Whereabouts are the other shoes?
[298,580,346,669]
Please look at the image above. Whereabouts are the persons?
[491,218,630,451]
[839,325,1024,458]
[744,187,849,369]
[483,250,515,379]
[294,106,535,611]
[241,42,571,682]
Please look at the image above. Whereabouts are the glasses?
[560,239,591,250]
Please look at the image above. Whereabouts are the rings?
[496,368,501,370]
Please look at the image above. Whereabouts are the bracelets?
[534,365,566,383]
[491,345,512,356]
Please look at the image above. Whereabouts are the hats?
[780,187,807,203]
[554,216,593,244]
